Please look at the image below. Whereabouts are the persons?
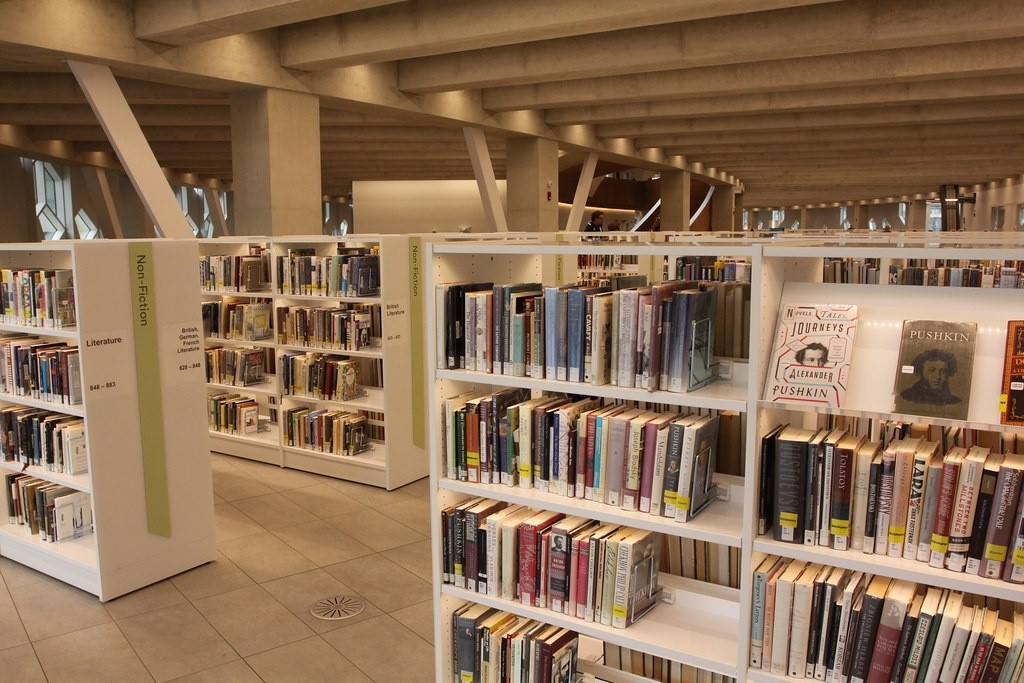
[559,655,570,683]
[583,210,604,232]
[902,348,963,404]
[550,536,565,552]
[795,342,829,366]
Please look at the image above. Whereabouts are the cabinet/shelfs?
[0,231,1024,683]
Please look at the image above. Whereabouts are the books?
[434,236,1024,683]
[1,267,93,544]
[197,245,385,458]
